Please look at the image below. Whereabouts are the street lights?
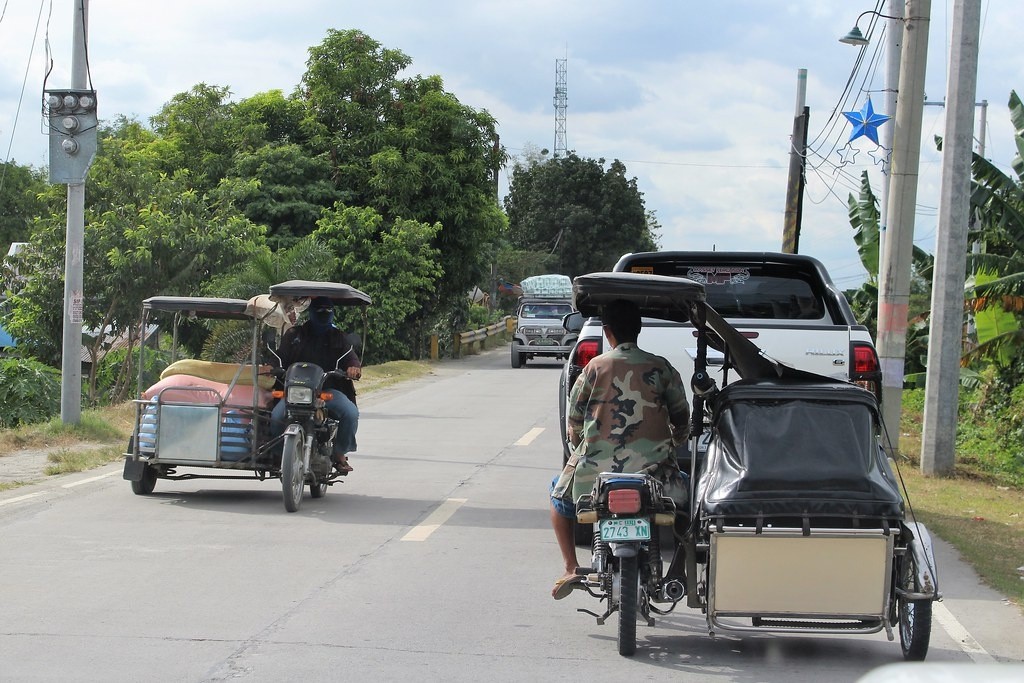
[837,11,931,459]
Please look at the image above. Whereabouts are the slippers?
[552,575,582,600]
[334,459,353,472]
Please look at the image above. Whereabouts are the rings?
[355,370,356,371]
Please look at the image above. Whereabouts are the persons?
[268,294,362,471]
[550,294,696,599]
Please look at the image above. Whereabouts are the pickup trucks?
[560,252,884,470]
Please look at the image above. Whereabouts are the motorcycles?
[122,279,373,512]
[568,271,944,662]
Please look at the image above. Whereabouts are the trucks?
[511,293,579,369]
